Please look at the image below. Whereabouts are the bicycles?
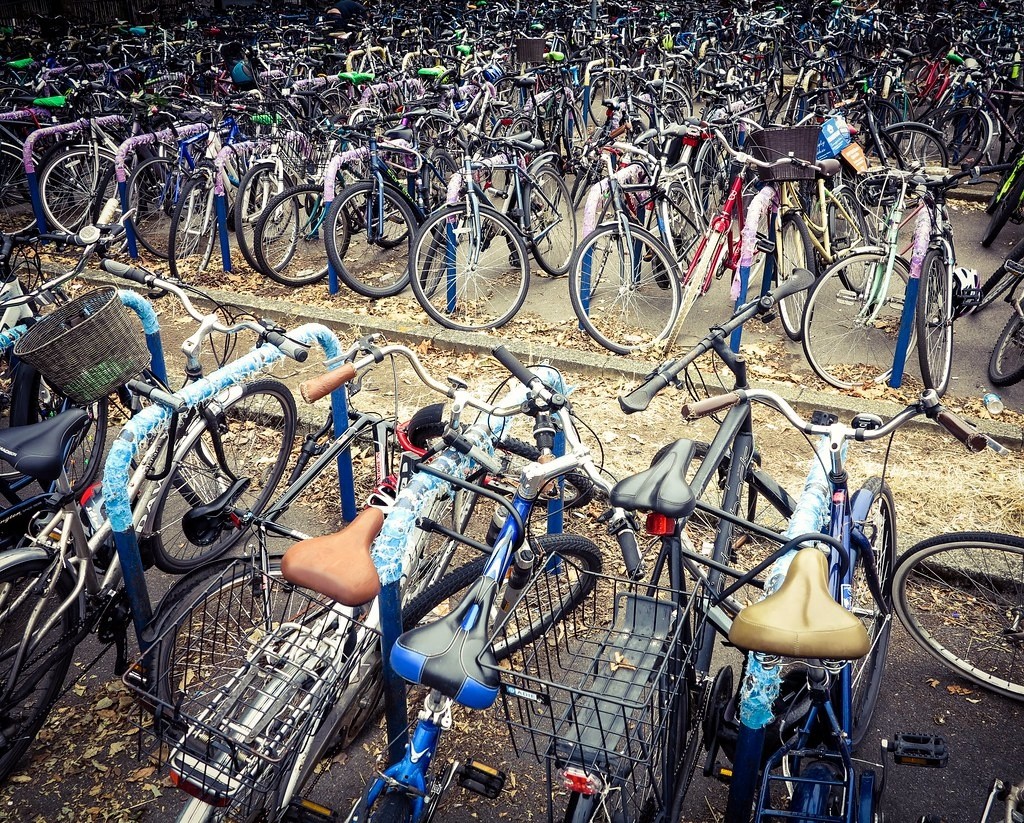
[1,0,1024,822]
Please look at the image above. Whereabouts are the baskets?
[14,285,151,405]
[475,551,705,788]
[516,38,546,63]
[223,100,331,180]
[749,125,823,181]
[124,558,384,823]
[135,58,186,91]
